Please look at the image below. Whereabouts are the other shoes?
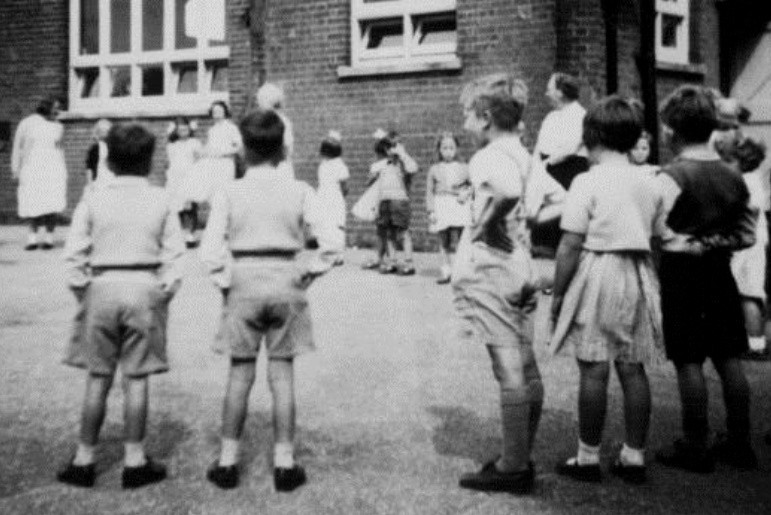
[556,456,601,481]
[437,275,450,282]
[274,464,307,492]
[402,266,416,274]
[608,457,646,484]
[42,242,54,248]
[25,243,38,249]
[658,435,700,470]
[743,348,769,360]
[459,457,535,493]
[703,431,754,468]
[121,455,167,489]
[381,264,397,273]
[363,259,381,267]
[58,455,97,487]
[206,458,239,489]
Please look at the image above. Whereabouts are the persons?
[450,74,570,494]
[200,110,344,492]
[256,83,295,181]
[630,84,771,473]
[533,71,589,295]
[553,94,664,480]
[11,97,69,250]
[317,132,350,265]
[364,129,419,275]
[165,102,245,249]
[85,118,114,182]
[426,133,474,284]
[56,123,185,489]
